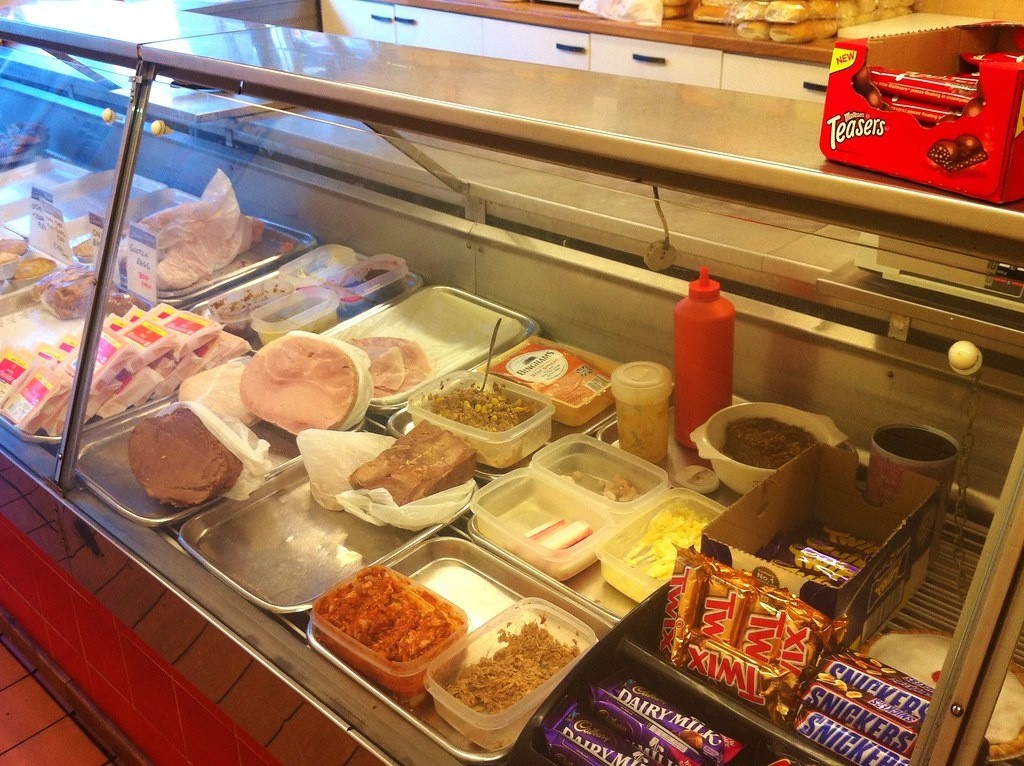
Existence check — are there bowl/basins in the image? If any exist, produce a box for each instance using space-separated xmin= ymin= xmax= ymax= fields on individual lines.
xmin=308 ymin=370 xmax=849 ymax=750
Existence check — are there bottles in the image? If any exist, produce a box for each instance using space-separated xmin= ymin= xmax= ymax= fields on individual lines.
xmin=673 ymin=264 xmax=737 ymax=449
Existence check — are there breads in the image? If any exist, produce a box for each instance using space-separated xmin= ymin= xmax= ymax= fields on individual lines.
xmin=659 ymin=541 xmax=833 ymax=729
xmin=660 ymin=0 xmax=916 ymax=44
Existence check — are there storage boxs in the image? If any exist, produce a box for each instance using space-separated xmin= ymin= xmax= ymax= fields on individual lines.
xmin=700 ymin=441 xmax=948 ymax=651
xmin=820 ymin=21 xmax=1024 ymax=205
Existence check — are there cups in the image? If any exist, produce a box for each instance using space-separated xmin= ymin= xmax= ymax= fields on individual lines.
xmin=864 ymin=422 xmax=960 ymax=520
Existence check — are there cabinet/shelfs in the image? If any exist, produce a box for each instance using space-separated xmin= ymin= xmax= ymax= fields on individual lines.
xmin=320 ymin=0 xmax=831 ymax=101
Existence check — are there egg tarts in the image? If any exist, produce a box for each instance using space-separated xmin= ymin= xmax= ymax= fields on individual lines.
xmin=71 ymin=238 xmax=94 ymax=263
xmin=0 ymin=238 xmax=56 ymax=290
